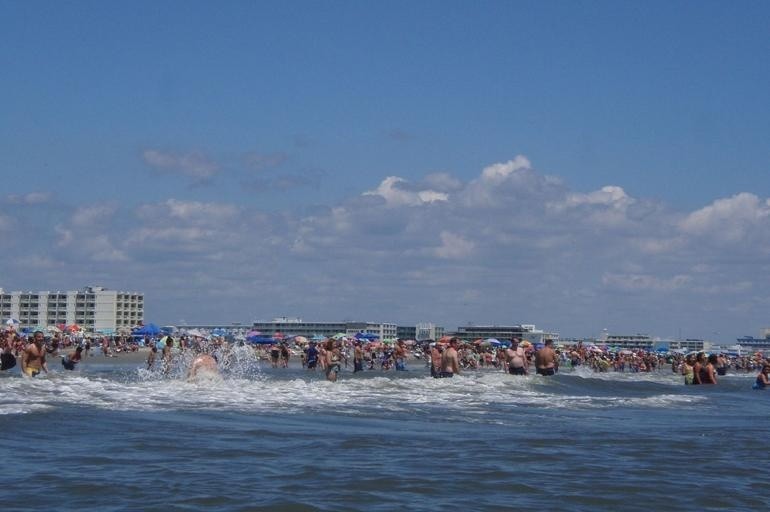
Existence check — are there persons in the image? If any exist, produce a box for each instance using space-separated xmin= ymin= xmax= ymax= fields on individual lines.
xmin=102 ymin=333 xmax=139 ymax=357
xmin=254 ymin=335 xmax=770 ymax=390
xmin=1 ymin=329 xmax=109 ymax=378
xmin=147 ymin=334 xmax=244 ymax=377
